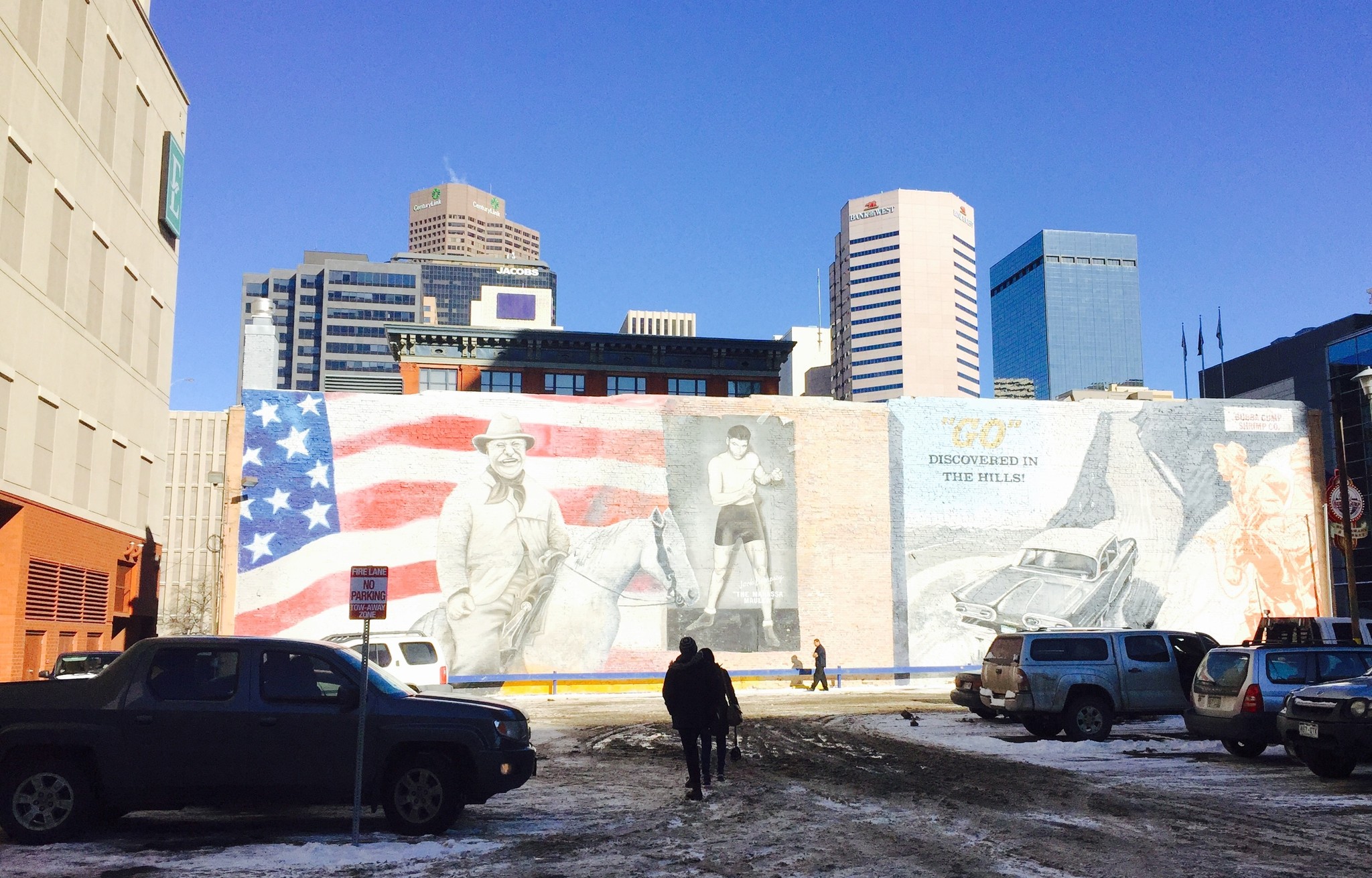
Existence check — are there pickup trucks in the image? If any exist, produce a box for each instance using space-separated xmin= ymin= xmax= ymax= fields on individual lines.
xmin=978 ymin=624 xmax=1221 ymax=741
xmin=1254 ymin=609 xmax=1372 ymax=644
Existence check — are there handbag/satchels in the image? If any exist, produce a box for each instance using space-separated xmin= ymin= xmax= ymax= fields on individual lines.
xmin=726 ymin=703 xmax=743 ymax=726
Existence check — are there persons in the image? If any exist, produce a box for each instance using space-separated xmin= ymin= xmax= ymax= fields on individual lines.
xmin=806 ymin=639 xmax=829 ymax=691
xmin=697 ymin=647 xmax=741 ymax=787
xmin=662 ymin=637 xmax=722 ymax=801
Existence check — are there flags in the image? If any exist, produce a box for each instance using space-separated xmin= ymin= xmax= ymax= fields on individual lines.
xmin=1197 ymin=320 xmax=1204 ymax=356
xmin=1181 ymin=330 xmax=1187 ymax=361
xmin=1216 ymin=310 xmax=1224 ymax=351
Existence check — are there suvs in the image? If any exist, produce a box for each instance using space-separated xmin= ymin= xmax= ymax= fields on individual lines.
xmin=319 ymin=630 xmax=452 ymax=692
xmin=1277 ymin=666 xmax=1372 ymax=777
xmin=1184 ymin=640 xmax=1372 ymax=757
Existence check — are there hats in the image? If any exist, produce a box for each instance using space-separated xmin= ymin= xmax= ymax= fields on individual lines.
xmin=471 ymin=421 xmax=536 ymax=454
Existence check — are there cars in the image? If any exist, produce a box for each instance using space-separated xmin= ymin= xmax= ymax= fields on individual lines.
xmin=38 ymin=651 xmax=126 ymax=682
xmin=950 ymin=668 xmax=1023 ymax=723
xmin=0 ymin=637 xmax=538 ymax=839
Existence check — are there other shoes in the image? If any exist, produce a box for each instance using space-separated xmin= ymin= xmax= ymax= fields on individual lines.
xmin=685 ymin=788 xmax=702 ymax=801
xmin=685 ymin=607 xmax=717 ymax=631
xmin=762 ymin=619 xmax=781 ymax=647
xmin=808 ymin=688 xmax=814 ymax=691
xmin=822 ymin=687 xmax=829 ymax=691
xmin=702 ymin=777 xmax=711 ymax=785
xmin=685 ymin=778 xmax=701 ymax=788
xmin=717 ymin=771 xmax=725 ymax=781
